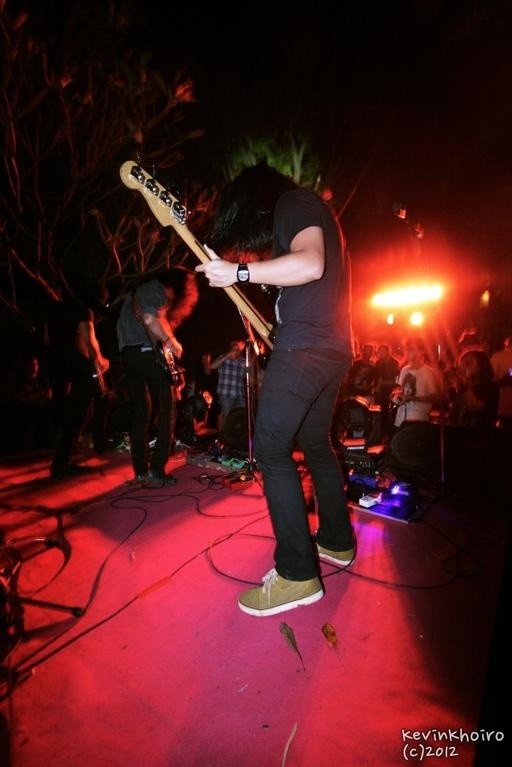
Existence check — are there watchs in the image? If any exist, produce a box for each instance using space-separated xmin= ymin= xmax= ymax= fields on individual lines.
xmin=237 ymin=263 xmax=249 ymax=283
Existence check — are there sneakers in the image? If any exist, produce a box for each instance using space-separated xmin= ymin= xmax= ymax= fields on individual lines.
xmin=310 ymin=539 xmax=359 ymax=567
xmin=50 ymin=440 xmax=179 ymax=489
xmin=237 ymin=567 xmax=328 ymax=617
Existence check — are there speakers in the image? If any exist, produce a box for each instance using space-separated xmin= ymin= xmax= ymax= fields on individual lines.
xmin=384 ymin=420 xmax=440 ymax=478
xmin=221 ymin=406 xmax=249 ymax=452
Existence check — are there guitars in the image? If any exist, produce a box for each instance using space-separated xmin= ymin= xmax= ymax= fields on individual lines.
xmin=118 ymin=162 xmax=275 ymax=371
xmin=161 ymin=338 xmax=180 ymax=385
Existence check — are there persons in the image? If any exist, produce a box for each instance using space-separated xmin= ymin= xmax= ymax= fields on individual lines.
xmin=48 ymin=281 xmax=113 ymax=480
xmin=192 ymin=165 xmax=357 ymax=619
xmin=112 ymin=264 xmax=200 ymax=487
